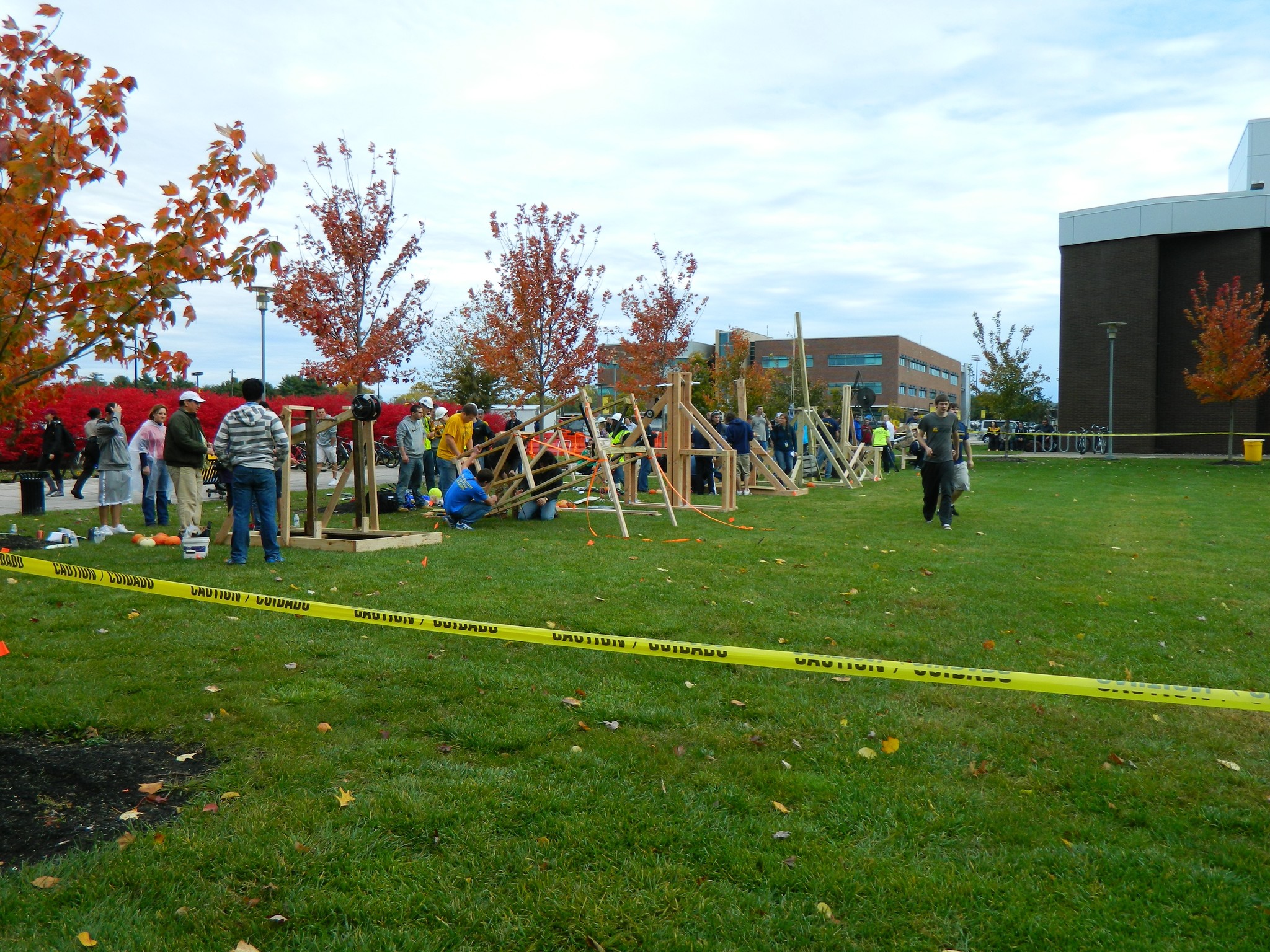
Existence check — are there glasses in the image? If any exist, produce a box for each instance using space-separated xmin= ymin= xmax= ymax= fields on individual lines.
xmin=100 ymin=412 xmax=103 ymax=414
xmin=779 ymin=418 xmax=785 ymax=419
xmin=712 ymin=411 xmax=720 ymax=416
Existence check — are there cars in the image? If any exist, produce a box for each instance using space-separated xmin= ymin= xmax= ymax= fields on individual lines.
xmin=1018 ymin=420 xmax=1042 ymax=434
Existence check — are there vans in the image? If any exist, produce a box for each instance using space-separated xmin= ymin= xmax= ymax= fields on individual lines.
xmin=977 ymin=420 xmax=1022 ymax=444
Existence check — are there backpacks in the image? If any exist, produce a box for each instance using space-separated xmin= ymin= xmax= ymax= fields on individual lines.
xmin=53 ymin=419 xmax=76 ymax=453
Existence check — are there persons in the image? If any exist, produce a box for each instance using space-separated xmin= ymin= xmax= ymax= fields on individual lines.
xmin=70 ymin=406 xmax=108 ymax=499
xmin=999 ymin=419 xmax=1014 ymax=452
xmin=312 ymin=407 xmax=340 ymax=487
xmin=93 ymin=402 xmax=136 ymax=535
xmin=987 ymin=421 xmax=1001 ymax=452
xmin=916 ymin=393 xmax=961 ymax=531
xmin=137 ymin=403 xmax=171 ymax=528
xmin=212 ymin=376 xmax=292 ymax=566
xmin=937 ymin=403 xmax=974 ymax=517
xmin=1033 ymin=417 xmax=1056 ymax=452
xmin=39 ymin=407 xmax=67 ymax=497
xmin=163 ymin=390 xmax=216 ymax=540
xmin=383 ymin=389 xmax=936 ymax=530
xmin=1015 ymin=421 xmax=1027 ymax=452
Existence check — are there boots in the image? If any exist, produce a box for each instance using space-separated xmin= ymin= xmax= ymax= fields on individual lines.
xmin=45 ymin=475 xmax=64 ymax=497
xmin=71 ymin=479 xmax=84 ymax=499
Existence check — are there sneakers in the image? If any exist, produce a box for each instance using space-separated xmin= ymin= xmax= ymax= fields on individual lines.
xmin=925 ymin=519 xmax=933 ymax=524
xmin=247 ymin=522 xmax=261 ymax=531
xmin=226 ymin=559 xmax=246 ymax=567
xmin=421 ymin=505 xmax=433 ymax=510
xmin=100 ymin=523 xmax=135 ymax=536
xmin=736 ymin=488 xmax=753 ymax=496
xmin=942 ymin=524 xmax=953 ymax=531
xmin=709 ymin=492 xmax=719 ymax=496
xmin=266 ymin=556 xmax=285 ymax=563
xmin=328 ymin=478 xmax=339 ymax=486
xmin=445 ymin=515 xmax=476 ymax=530
xmin=397 ymin=507 xmax=409 ymax=512
xmin=937 ymin=505 xmax=959 ymax=517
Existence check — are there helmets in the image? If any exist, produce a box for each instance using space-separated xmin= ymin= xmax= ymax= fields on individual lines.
xmin=598 ymin=413 xmax=625 ymax=423
xmin=419 ymin=396 xmax=448 ymax=420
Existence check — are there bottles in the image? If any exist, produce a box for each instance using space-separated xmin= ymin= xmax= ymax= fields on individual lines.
xmin=294 ymin=514 xmax=300 ymax=528
xmin=88 ymin=526 xmax=105 ymax=543
xmin=70 ymin=531 xmax=79 ymax=547
xmin=11 ymin=524 xmax=18 ymax=535
xmin=584 ymin=479 xmax=590 ymax=489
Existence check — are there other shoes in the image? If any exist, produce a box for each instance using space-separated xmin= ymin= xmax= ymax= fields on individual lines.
xmin=638 ymin=489 xmax=650 ymax=492
xmin=148 ymin=522 xmax=172 ymax=528
xmin=422 ymin=471 xmax=440 ymax=477
xmin=906 ymin=461 xmax=921 ymax=470
xmin=987 ymin=449 xmax=1027 ymax=452
xmin=177 ymin=532 xmax=197 ymax=540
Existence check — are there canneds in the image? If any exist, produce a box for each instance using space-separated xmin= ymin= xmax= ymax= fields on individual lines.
xmin=61 ymin=534 xmax=69 ymax=543
xmin=36 ymin=530 xmax=43 ymax=539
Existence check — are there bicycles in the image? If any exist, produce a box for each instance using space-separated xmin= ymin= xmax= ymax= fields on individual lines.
xmin=280 ymin=434 xmax=402 ymax=471
xmin=71 ymin=435 xmax=91 ymax=480
xmin=1076 ymin=426 xmax=1091 ymax=455
xmin=1091 ymin=423 xmax=1110 ymax=455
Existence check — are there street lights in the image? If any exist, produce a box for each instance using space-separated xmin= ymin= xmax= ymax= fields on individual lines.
xmin=1099 ymin=317 xmax=1125 ymax=458
xmin=611 ymin=356 xmax=620 ymax=418
xmin=245 ymin=282 xmax=282 ymax=403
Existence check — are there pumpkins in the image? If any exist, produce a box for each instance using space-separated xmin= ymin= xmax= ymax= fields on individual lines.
xmin=132 ymin=532 xmax=169 ymax=548
xmin=807 ymin=481 xmax=815 ymax=487
xmin=656 ymin=489 xmax=663 ymax=494
xmin=165 ymin=535 xmax=182 ymax=546
xmin=555 ymin=499 xmax=573 ymax=508
xmin=649 ymin=489 xmax=656 ymax=494
xmin=605 ymin=486 xmax=609 ymax=492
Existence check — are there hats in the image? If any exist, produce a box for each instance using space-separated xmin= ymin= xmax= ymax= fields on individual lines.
xmin=40 ymin=407 xmax=58 ymax=415
xmin=776 ymin=412 xmax=782 ymax=418
xmin=477 ymin=409 xmax=485 ymax=415
xmin=180 ymin=391 xmax=206 ymax=402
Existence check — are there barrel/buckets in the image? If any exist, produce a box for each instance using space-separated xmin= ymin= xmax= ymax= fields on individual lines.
xmin=1243 ymin=439 xmax=1263 ymax=461
xmin=180 ymin=537 xmax=210 ymax=559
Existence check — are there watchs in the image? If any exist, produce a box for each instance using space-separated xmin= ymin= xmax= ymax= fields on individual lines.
xmin=1047 ymin=436 xmax=1049 ymax=439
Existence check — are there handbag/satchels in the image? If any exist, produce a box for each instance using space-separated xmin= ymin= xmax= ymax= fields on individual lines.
xmin=365 ymin=488 xmax=398 ymax=515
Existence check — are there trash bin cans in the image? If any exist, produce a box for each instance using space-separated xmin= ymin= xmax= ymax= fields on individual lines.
xmin=16 ymin=472 xmax=50 ymax=514
